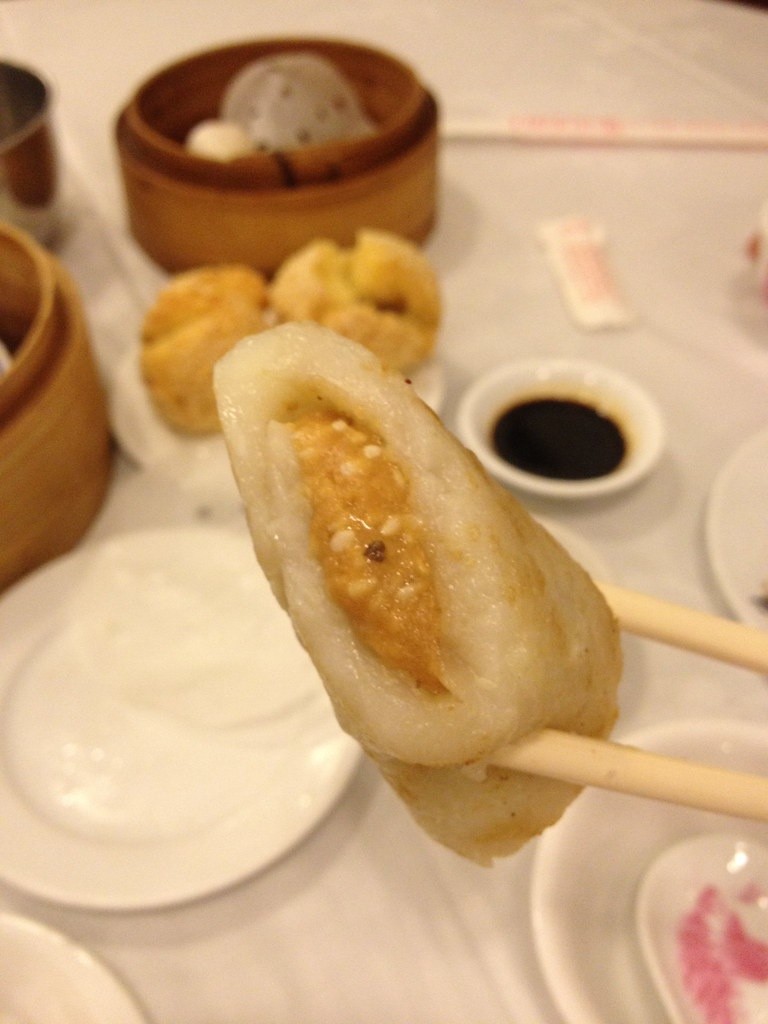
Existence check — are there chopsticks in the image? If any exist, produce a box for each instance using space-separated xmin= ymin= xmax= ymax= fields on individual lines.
xmin=491 ymin=579 xmax=766 ymax=822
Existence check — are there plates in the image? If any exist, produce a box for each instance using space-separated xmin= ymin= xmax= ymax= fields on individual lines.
xmin=1 ymin=526 xmax=365 ymax=910
xmin=2 ymin=911 xmax=146 ymax=1024
xmin=528 ymin=720 xmax=768 ymax=1024
xmin=708 ymin=427 xmax=767 ymax=634
xmin=109 ymin=321 xmax=443 ymax=498
xmin=459 ymin=357 xmax=668 ymax=500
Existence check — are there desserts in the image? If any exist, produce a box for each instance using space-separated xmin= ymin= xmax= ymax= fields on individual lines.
xmin=138 ymin=225 xmax=444 ymax=443
